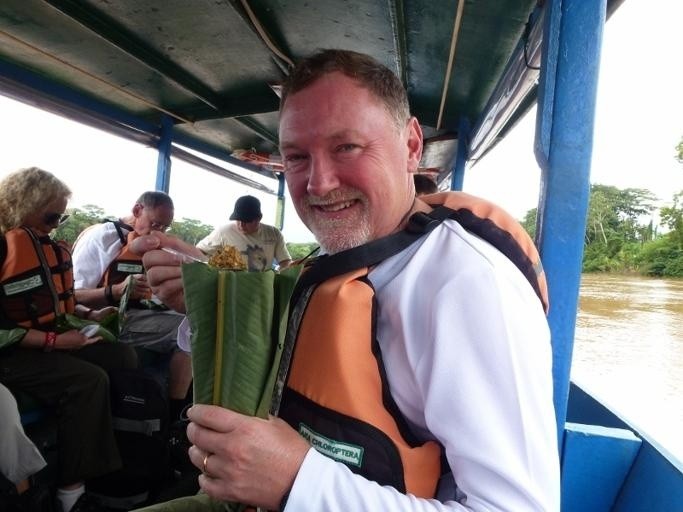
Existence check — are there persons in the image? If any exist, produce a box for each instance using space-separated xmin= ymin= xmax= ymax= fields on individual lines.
xmin=73 ymin=190 xmax=193 ymax=457
xmin=186 ymin=51 xmax=560 ymax=512
xmin=195 ymin=195 xmax=292 ymax=274
xmin=0 ymin=167 xmax=132 ymax=512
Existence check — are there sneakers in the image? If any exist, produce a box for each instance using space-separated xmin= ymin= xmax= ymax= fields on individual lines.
xmin=15 ymin=487 xmax=149 ymax=512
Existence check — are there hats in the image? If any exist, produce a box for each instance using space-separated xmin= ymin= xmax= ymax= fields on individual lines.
xmin=230 ymin=195 xmax=260 ymax=220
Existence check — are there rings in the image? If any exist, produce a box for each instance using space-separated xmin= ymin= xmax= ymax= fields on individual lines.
xmin=204 ymin=453 xmax=215 ymax=474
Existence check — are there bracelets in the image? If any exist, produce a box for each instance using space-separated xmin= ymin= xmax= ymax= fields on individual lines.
xmin=105 ymin=285 xmax=115 ymax=302
xmin=84 ymin=309 xmax=94 ymax=319
xmin=44 ymin=331 xmax=56 ymax=348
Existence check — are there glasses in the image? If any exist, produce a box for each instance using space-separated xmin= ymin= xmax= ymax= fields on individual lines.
xmin=152 ymin=223 xmax=172 ymax=234
xmin=43 ymin=213 xmax=70 ymax=225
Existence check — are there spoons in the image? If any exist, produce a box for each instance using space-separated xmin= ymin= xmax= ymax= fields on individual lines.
xmin=157 ymin=243 xmax=250 ymax=272
